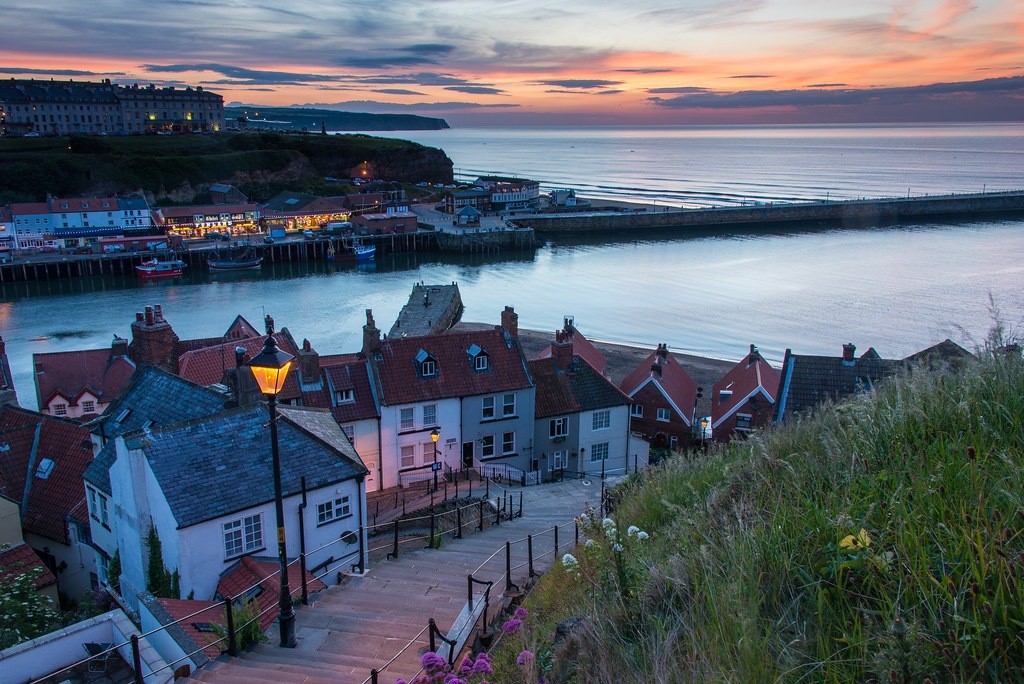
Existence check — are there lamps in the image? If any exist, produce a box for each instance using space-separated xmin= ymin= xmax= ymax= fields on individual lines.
xmin=82 ymin=440 xmax=97 ymax=451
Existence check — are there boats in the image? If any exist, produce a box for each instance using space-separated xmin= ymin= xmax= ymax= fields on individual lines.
xmin=353 ymin=245 xmax=376 ymax=265
xmin=134 ymin=253 xmax=188 ymax=276
xmin=205 ymin=233 xmax=264 ymax=269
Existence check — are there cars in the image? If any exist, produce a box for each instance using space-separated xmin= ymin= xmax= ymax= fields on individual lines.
xmin=324 ymin=176 xmax=337 ymax=182
xmin=416 ymin=182 xmax=428 ymax=187
xmin=433 ymin=183 xmax=491 ymax=192
xmin=350 ymin=177 xmax=366 ymax=186
xmin=204 ymin=232 xmax=221 ymax=240
xmin=303 ymin=228 xmax=313 ymax=235
xmin=263 ymin=236 xmax=274 ymax=244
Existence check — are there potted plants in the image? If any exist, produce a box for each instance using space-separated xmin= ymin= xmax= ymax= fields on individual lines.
xmin=554 ymin=435 xmax=566 ymax=443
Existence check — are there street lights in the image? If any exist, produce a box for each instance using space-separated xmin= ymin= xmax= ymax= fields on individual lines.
xmin=429 ymin=428 xmax=440 ymax=491
xmin=245 ymin=325 xmax=296 ymax=648
xmin=701 ymin=416 xmax=710 ymax=445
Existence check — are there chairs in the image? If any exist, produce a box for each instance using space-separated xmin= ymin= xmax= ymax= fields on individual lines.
xmin=82 ymin=642 xmax=120 ymax=673
